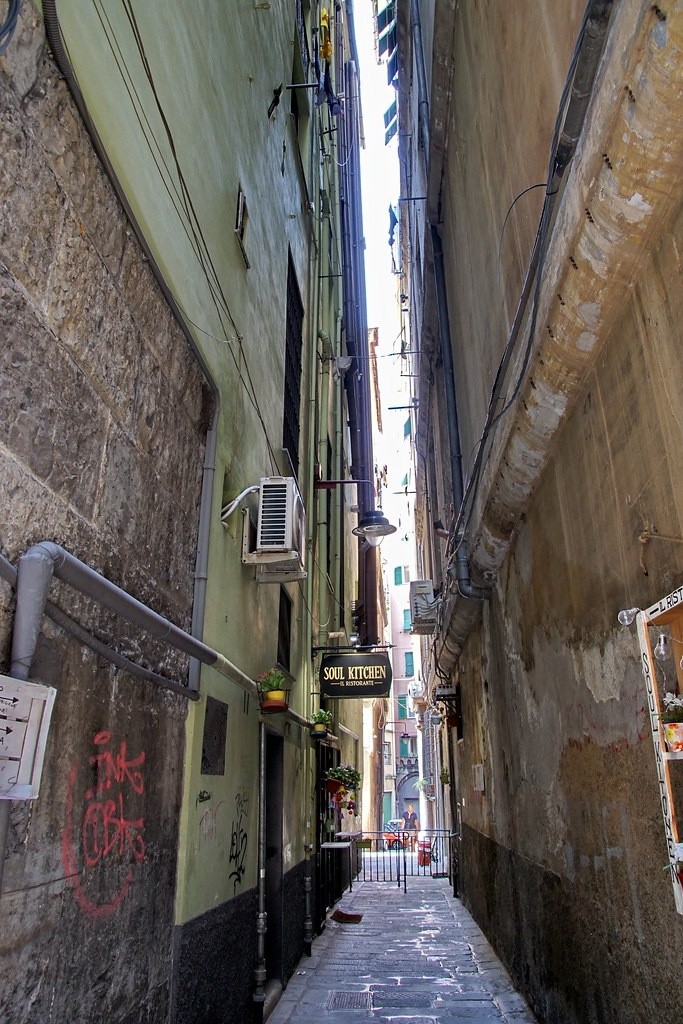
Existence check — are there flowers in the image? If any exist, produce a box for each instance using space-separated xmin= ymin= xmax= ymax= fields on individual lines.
xmin=326 ymin=762 xmax=362 ymax=819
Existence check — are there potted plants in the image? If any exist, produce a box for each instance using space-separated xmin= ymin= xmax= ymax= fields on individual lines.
xmin=256 ymin=667 xmax=293 ymax=701
xmin=440 ymin=767 xmax=449 ymax=783
xmin=309 ymin=709 xmax=334 ymax=731
xmin=430 ymin=711 xmax=441 ymax=725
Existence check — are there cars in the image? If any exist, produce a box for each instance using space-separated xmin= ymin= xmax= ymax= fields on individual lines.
xmin=388 ymin=819 xmax=406 ymax=829
xmin=384 ymin=824 xmax=408 ymax=848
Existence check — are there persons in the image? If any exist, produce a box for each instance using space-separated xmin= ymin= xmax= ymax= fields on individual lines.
xmin=400 ymin=804 xmax=419 ymax=853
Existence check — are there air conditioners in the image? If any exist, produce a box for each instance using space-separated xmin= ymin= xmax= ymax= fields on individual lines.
xmin=256 ymin=476 xmax=307 ymax=571
xmin=410 ymin=579 xmax=437 ymax=632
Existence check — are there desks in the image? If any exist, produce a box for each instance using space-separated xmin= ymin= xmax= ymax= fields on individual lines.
xmin=322 ymin=842 xmax=353 ymax=894
xmin=335 ymin=831 xmax=361 ymax=836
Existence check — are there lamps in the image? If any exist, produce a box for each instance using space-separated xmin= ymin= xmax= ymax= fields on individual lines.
xmin=317 ymin=480 xmax=397 ymax=547
xmin=352 ymin=639 xmax=361 ymax=647
xmin=385 ymin=721 xmax=413 ymax=744
xmin=349 ymin=632 xmax=358 ymax=641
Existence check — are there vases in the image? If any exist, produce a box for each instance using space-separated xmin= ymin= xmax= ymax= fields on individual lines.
xmin=429 ymin=784 xmax=434 ymax=792
xmin=328 ymin=778 xmax=342 ymax=794
